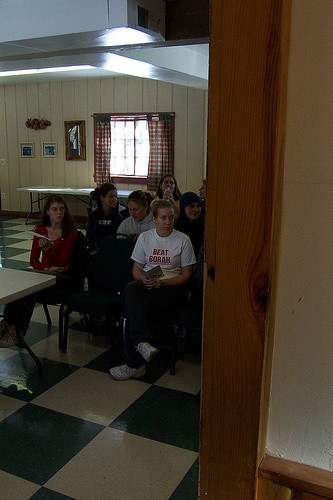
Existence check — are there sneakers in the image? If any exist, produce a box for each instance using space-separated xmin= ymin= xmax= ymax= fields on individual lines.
xmin=110 ymin=364 xmax=145 ymax=380
xmin=0 ymin=325 xmax=22 ymax=347
xmin=0 ymin=320 xmax=10 ymax=337
xmin=134 ymin=342 xmax=159 ymax=362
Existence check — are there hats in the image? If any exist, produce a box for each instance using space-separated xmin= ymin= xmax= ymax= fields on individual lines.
xmin=180 ymin=192 xmax=200 ymax=210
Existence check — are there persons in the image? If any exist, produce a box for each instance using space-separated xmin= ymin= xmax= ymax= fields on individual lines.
xmin=0 ymin=196 xmax=79 ymax=347
xmin=117 ymin=189 xmax=157 ymax=243
xmin=150 ymin=175 xmax=182 ymax=218
xmin=173 ymin=192 xmax=205 ymax=319
xmin=109 ymin=200 xmax=197 ymax=381
xmin=199 ymin=179 xmax=206 ymax=198
xmin=86 ymin=182 xmax=130 ymax=255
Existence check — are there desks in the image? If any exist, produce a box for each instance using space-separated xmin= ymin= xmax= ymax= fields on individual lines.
xmin=16 ymin=187 xmax=140 ymax=225
xmin=0 ymin=266 xmax=56 ymax=378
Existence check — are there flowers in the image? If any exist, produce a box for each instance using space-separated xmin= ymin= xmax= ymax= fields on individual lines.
xmin=25 ymin=117 xmax=51 ymax=130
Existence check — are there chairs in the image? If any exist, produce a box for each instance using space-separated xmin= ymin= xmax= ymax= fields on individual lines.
xmin=34 ymin=229 xmax=197 ymax=354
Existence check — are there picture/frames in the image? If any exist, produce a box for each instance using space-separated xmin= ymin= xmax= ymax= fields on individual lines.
xmin=41 ymin=141 xmax=57 ymax=158
xmin=65 ymin=120 xmax=85 ymax=160
xmin=19 ymin=142 xmax=34 ymax=157
